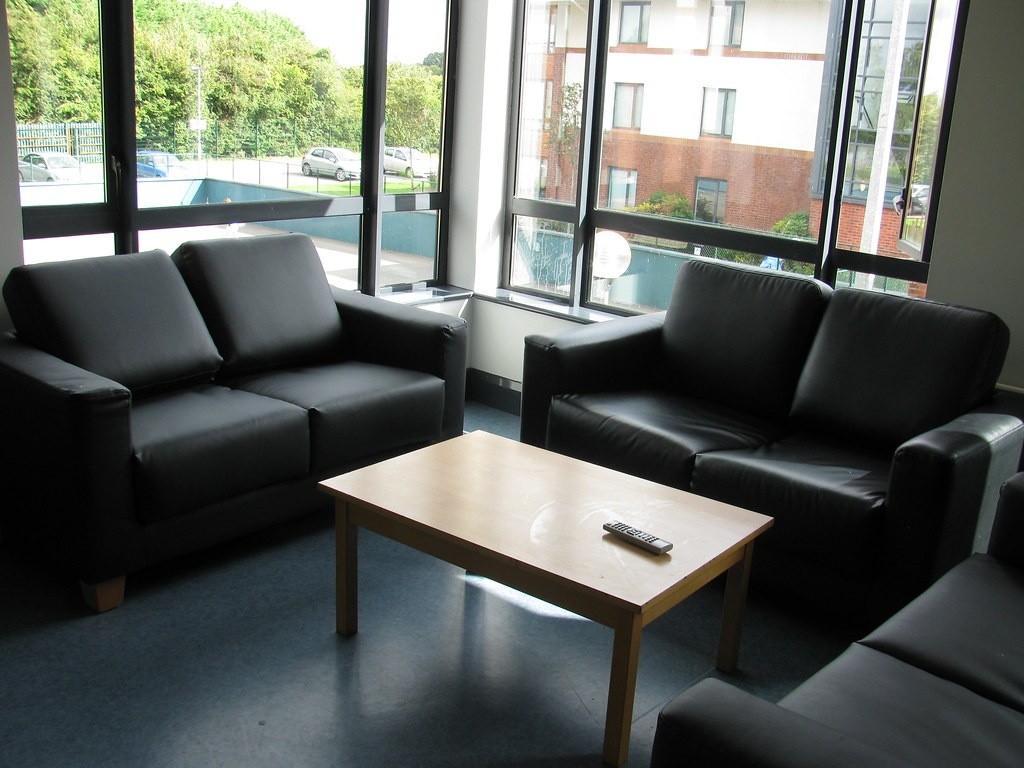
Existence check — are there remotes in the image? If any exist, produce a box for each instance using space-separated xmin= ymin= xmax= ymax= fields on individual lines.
xmin=603 ymin=520 xmax=673 ymax=556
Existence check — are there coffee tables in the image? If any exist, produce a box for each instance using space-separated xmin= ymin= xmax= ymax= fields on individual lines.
xmin=316 ymin=429 xmax=776 ymax=768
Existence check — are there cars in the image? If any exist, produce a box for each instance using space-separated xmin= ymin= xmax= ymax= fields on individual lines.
xmin=136 ymin=151 xmax=186 ymax=179
xmin=19 ymin=153 xmax=88 ymax=181
xmin=902 ymin=183 xmax=930 ymax=215
xmin=381 ymin=146 xmax=439 ymax=179
xmin=300 ymin=147 xmax=365 ymax=181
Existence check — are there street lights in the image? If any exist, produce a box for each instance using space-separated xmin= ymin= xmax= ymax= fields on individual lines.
xmin=189 ymin=65 xmax=206 ymax=159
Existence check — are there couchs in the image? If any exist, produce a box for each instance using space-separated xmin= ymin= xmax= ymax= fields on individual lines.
xmin=519 ymin=255 xmax=1024 ymax=632
xmin=648 ymin=471 xmax=1024 ymax=768
xmin=0 ymin=232 xmax=468 ymax=614
